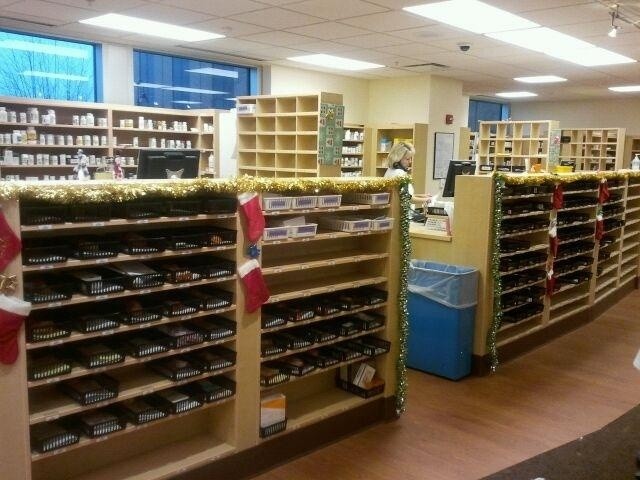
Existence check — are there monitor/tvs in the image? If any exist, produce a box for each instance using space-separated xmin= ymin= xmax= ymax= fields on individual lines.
xmin=136 ymin=147 xmax=200 ymax=180
xmin=442 ymin=160 xmax=476 ymax=198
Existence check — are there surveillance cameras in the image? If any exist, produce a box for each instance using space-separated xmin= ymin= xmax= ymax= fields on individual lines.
xmin=458 ymin=43 xmax=471 ymax=52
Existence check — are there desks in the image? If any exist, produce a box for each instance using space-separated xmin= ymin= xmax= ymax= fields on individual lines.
xmin=408 ymin=217 xmax=453 ymax=264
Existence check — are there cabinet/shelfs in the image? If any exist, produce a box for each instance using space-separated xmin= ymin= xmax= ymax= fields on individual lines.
xmin=580 ymin=132 xmax=640 ymax=171
xmin=0 ymin=96 xmax=229 ymax=182
xmin=340 ymin=123 xmax=374 ymax=177
xmin=520 ymin=135 xmax=544 ymax=169
xmin=235 ymin=92 xmax=345 ymax=178
xmin=372 ymin=122 xmax=428 ymax=209
xmin=543 ymin=128 xmax=634 ymax=172
xmin=458 ymin=127 xmax=512 ymax=171
xmin=0 ymin=176 xmax=403 ymax=480
xmin=476 ymin=120 xmax=561 ymax=177
xmin=445 ymin=170 xmax=640 ymax=356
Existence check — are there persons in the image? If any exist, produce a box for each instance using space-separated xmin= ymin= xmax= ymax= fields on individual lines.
xmin=385 ymin=141 xmax=432 ymax=220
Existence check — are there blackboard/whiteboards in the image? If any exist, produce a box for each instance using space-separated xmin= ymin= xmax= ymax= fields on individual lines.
xmin=434 ymin=131 xmax=455 ymax=180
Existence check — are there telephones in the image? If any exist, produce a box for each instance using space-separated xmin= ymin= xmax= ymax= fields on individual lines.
xmin=393 ymin=162 xmax=428 ymax=225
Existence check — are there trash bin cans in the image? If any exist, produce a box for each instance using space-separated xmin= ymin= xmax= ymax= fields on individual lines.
xmin=406 ymin=260 xmax=479 ymax=382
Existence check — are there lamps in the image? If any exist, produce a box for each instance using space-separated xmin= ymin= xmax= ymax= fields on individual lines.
xmin=608 ymin=16 xmax=620 ymax=40
